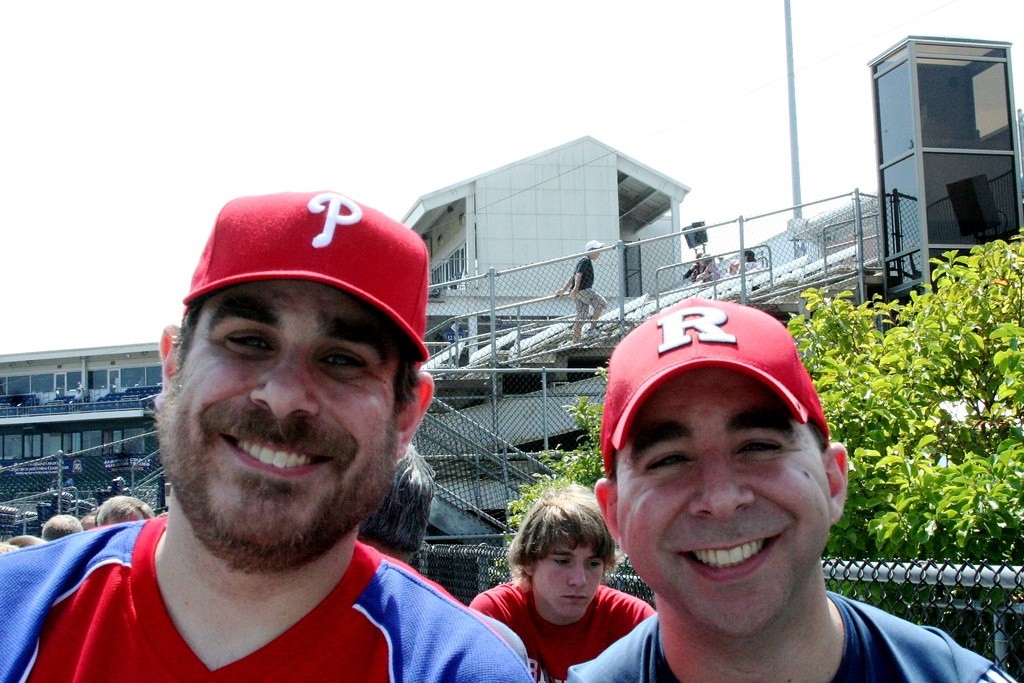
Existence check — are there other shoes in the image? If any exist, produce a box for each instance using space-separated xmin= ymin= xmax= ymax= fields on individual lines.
xmin=587 ymin=328 xmax=601 ymax=334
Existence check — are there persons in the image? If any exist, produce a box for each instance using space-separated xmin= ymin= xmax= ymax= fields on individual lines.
xmin=467 ymin=483 xmax=660 ymax=682
xmin=0 ymin=189 xmax=539 ymax=682
xmin=357 ymin=444 xmax=436 ymax=565
xmin=681 ymin=249 xmax=760 ymax=285
xmin=68 ymin=388 xmax=84 ymax=411
xmin=0 ymin=473 xmax=159 ymax=557
xmin=562 ymin=297 xmax=1016 ymax=682
xmin=555 ymin=240 xmax=607 ymax=346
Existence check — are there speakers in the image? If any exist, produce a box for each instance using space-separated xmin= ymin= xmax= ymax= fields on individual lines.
xmin=945 ymin=173 xmax=1002 ymax=236
xmin=682 ymin=222 xmax=707 ymax=249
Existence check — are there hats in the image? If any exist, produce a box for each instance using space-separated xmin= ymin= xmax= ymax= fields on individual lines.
xmin=182 ymin=190 xmax=431 ymax=371
xmin=586 ymin=240 xmax=605 ymax=252
xmin=599 ymin=299 xmax=829 ymax=480
